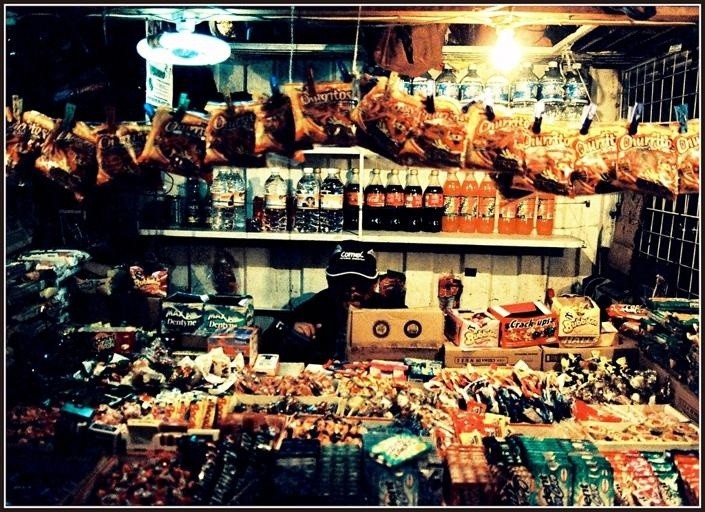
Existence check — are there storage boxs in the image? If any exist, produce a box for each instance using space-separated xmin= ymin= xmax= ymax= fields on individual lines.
xmin=444 ymin=342 xmax=542 ymax=371
xmin=345 ymin=304 xmax=445 ymax=348
xmin=540 ymin=338 xmax=640 ymax=373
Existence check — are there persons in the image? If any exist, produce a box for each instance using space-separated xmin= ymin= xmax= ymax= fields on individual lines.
xmin=265 ymin=245 xmax=407 ymax=371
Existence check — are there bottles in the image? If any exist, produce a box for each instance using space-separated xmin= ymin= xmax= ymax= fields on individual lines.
xmin=261 ymin=166 xmax=345 ymax=234
xmin=347 ymin=167 xmax=557 ymax=232
xmin=226 ymin=168 xmax=246 ymax=228
xmin=399 ymin=61 xmax=592 ymax=117
xmin=213 ymin=168 xmax=233 ymax=231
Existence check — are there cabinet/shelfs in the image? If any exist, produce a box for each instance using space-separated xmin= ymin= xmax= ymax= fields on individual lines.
xmin=135 ymin=48 xmax=585 ymax=248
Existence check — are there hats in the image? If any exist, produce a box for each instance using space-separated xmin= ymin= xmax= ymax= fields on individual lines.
xmin=325 ymin=240 xmax=379 ymax=280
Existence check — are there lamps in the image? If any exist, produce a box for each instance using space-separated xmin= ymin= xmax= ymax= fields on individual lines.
xmin=136 ymin=19 xmax=231 ymax=66
